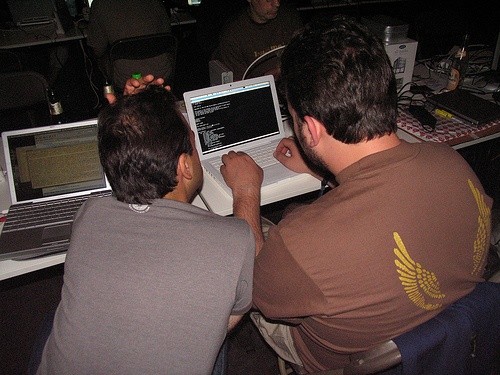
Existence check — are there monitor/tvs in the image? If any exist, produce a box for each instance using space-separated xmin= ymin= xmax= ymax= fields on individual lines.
xmin=87 ymin=0 xmax=93 ymax=9
xmin=187 ymin=0 xmax=202 ymax=7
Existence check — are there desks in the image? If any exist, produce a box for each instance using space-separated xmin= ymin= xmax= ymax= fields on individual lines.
xmin=0 ymin=60 xmax=500 ymax=281
xmin=170 ymin=12 xmax=196 ymax=26
xmin=295 ymin=0 xmax=396 ymax=11
xmin=0 ymin=15 xmax=85 ymax=50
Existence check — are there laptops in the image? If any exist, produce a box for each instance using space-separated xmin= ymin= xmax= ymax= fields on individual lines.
xmin=183 ymin=74 xmax=302 ymax=195
xmin=0 ymin=118 xmax=114 ymax=260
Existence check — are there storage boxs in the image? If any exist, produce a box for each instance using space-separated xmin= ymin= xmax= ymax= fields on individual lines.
xmin=383 ymin=37 xmax=418 ymax=98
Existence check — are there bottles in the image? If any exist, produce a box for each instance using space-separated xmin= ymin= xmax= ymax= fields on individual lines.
xmin=45 ymin=86 xmax=67 ymax=126
xmin=98 ymin=70 xmax=119 ymax=116
xmin=131 ymin=72 xmax=142 ymax=79
xmin=446 ymin=32 xmax=473 ymax=90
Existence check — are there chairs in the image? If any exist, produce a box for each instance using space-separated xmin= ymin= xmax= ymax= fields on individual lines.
xmin=414 ymin=11 xmax=454 ymax=59
xmin=278 ymin=272 xmax=500 ymax=375
xmin=100 ymin=33 xmax=178 ymax=98
xmin=0 ymin=70 xmax=53 ymax=127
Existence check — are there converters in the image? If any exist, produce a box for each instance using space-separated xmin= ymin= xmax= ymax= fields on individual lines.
xmin=408 ymin=105 xmax=437 ymax=126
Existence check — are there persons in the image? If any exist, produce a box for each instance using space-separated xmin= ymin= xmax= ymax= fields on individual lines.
xmin=221 ymin=14 xmax=493 ymax=375
xmin=86 ymin=0 xmax=173 ymax=89
xmin=28 ymin=74 xmax=255 ymax=375
xmin=212 ymin=0 xmax=307 ymax=83
